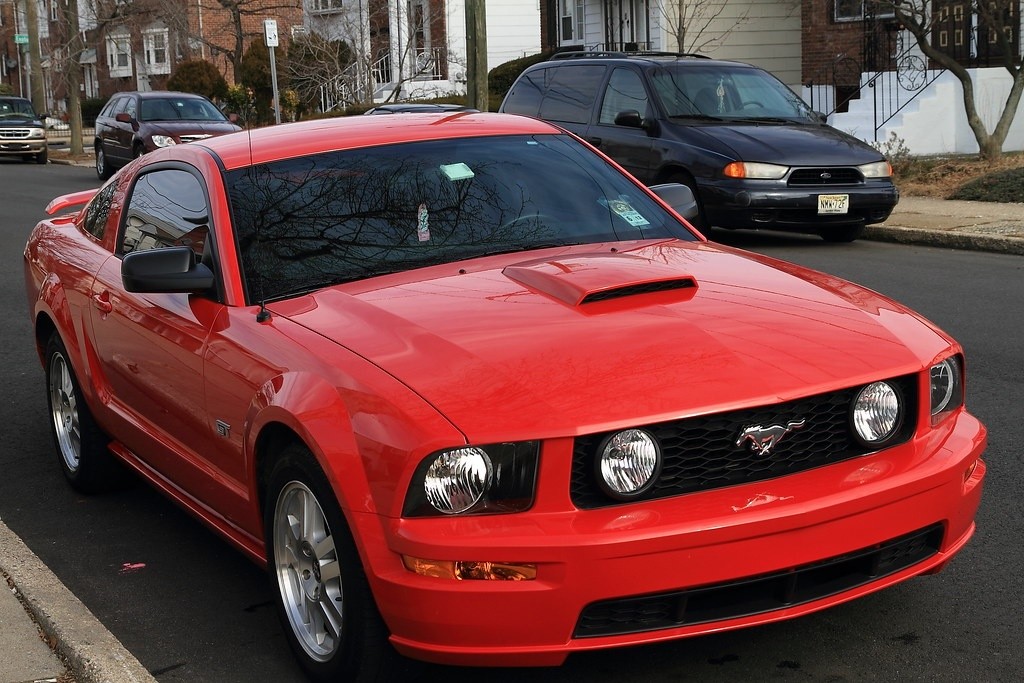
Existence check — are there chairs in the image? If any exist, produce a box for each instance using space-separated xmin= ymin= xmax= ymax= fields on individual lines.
xmin=692 ymin=88 xmax=721 ymax=115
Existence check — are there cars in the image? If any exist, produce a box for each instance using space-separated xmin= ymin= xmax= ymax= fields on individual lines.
xmin=365 ymin=102 xmax=482 ymax=115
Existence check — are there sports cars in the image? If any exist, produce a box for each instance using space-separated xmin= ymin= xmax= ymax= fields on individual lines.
xmin=22 ymin=110 xmax=990 ymax=683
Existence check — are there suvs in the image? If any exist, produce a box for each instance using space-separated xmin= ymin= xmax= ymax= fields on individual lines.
xmin=94 ymin=91 xmax=244 ymax=180
xmin=494 ymin=48 xmax=901 ymax=244
xmin=0 ymin=97 xmax=48 ymax=165
